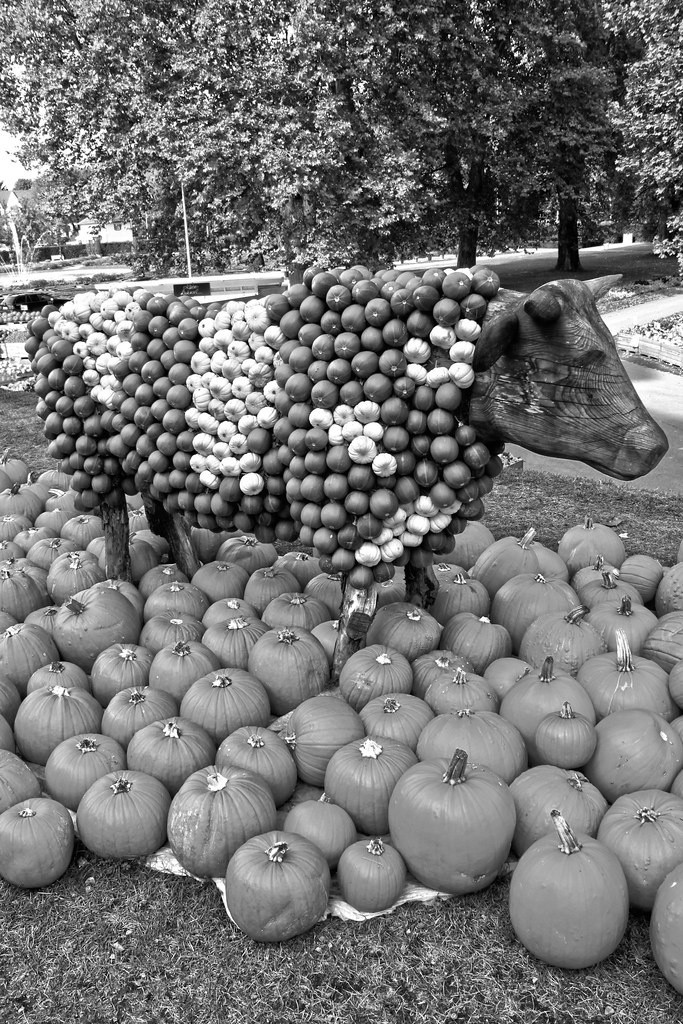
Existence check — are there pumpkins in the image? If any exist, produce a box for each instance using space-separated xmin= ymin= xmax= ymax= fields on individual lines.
xmin=0 ymin=264 xmax=683 ymax=995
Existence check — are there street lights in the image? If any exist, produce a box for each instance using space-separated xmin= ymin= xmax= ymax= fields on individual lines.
xmin=180 ymin=172 xmax=192 ymax=278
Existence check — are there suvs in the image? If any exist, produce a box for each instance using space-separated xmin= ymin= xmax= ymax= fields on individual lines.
xmin=2 ymin=292 xmax=72 ymax=314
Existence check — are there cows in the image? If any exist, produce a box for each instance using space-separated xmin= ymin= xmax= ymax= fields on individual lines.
xmin=26 ymin=275 xmax=668 ymax=610
